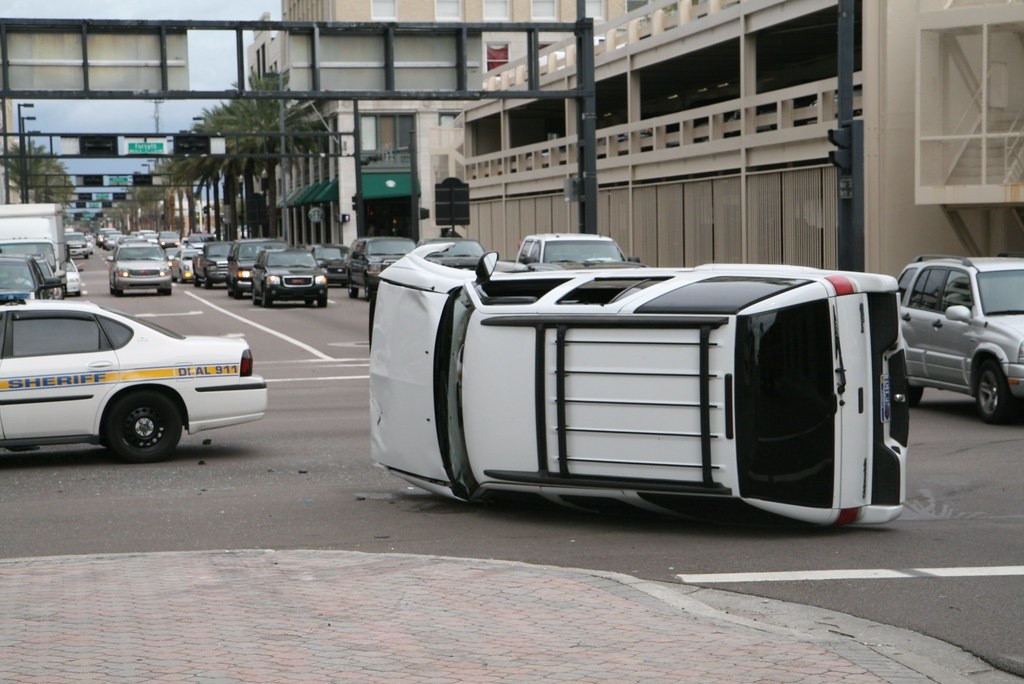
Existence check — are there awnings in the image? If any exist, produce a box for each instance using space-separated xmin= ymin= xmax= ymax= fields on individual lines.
xmin=276 ymin=177 xmax=340 ymax=208
xmin=360 ymin=172 xmax=421 ymax=199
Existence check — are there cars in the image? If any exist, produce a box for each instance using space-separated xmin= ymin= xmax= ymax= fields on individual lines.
xmin=417 ymin=239 xmax=487 ymax=269
xmin=0 ymin=292 xmax=270 ymax=461
xmin=170 ymin=249 xmax=200 ymax=283
xmin=107 ymin=244 xmax=175 ymax=297
xmin=304 ymin=245 xmax=351 ymax=285
xmin=33 ymin=254 xmax=65 ymax=300
xmin=89 ymin=228 xmax=219 ymax=258
xmin=66 ymin=258 xmax=85 ymax=295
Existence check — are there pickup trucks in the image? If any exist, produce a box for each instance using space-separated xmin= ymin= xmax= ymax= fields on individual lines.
xmin=192 ymin=242 xmax=235 ymax=289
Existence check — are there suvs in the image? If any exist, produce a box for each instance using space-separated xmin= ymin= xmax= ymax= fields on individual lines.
xmin=252 ymin=248 xmax=328 ymax=308
xmin=0 ymin=255 xmax=61 ymax=300
xmin=345 ymin=236 xmax=416 ymax=298
xmin=65 ymin=232 xmax=88 ymax=259
xmin=227 ymin=241 xmax=290 ymax=299
xmin=515 ymin=233 xmax=630 ymax=265
xmin=892 ymin=253 xmax=1024 ymax=423
xmin=367 ymin=239 xmax=911 ymax=528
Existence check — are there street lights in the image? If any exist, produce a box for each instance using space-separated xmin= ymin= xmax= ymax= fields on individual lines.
xmin=18 ymin=102 xmax=42 ymax=203
xmin=140 ymin=117 xmax=211 ymax=234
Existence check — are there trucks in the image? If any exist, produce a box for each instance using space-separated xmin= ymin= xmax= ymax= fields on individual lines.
xmin=0 ymin=203 xmax=68 ymax=294
